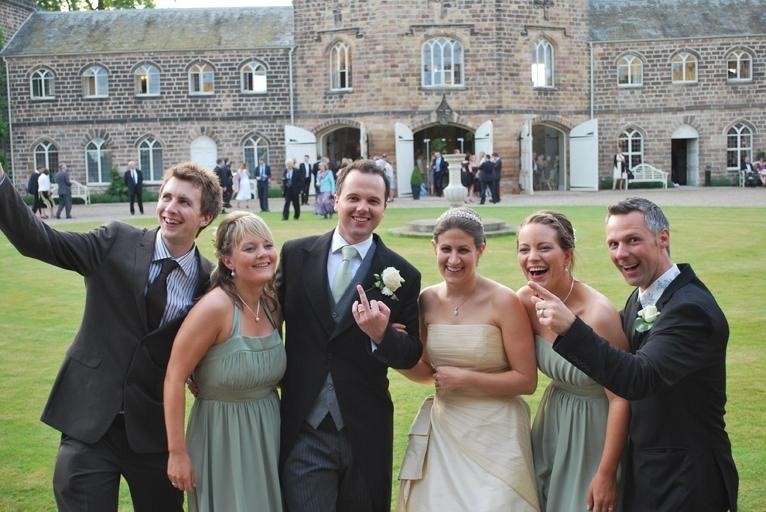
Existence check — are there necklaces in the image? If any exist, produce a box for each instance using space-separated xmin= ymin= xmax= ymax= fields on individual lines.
xmin=232 ymin=287 xmax=262 ymax=323
xmin=563 ymin=274 xmax=575 ymax=304
xmin=442 ymin=275 xmax=481 ymax=316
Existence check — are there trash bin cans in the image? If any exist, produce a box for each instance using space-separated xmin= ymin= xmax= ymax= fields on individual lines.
xmin=705 ymin=166 xmax=711 ymax=185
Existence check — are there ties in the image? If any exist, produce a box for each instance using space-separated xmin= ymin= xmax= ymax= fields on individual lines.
xmin=145 ymin=258 xmax=179 ymax=333
xmin=332 ymin=246 xmax=357 ymax=304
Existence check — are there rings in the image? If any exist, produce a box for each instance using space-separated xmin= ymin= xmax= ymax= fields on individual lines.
xmin=608 ymin=504 xmax=613 ymax=511
xmin=172 ymin=482 xmax=177 ymax=487
xmin=539 ymin=309 xmax=544 ymax=317
xmin=357 ymin=306 xmax=364 ymax=313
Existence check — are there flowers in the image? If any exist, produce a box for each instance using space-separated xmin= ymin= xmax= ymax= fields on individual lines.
xmin=632 ymin=302 xmax=663 ymax=339
xmin=362 ymin=264 xmax=406 ymax=302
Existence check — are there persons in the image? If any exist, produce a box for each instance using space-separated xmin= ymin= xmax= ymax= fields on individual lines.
xmin=511 ymin=209 xmax=629 ymax=512
xmin=526 ymin=196 xmax=739 ymax=512
xmin=0 ymin=161 xmax=219 ymax=512
xmin=389 ymin=207 xmax=542 ymax=512
xmin=612 ymin=148 xmax=627 ymax=191
xmin=186 ymin=158 xmax=423 ymax=512
xmin=161 ymin=209 xmax=287 ymax=512
xmin=741 ymin=155 xmax=762 ymax=187
xmin=756 ymin=157 xmax=766 ymax=187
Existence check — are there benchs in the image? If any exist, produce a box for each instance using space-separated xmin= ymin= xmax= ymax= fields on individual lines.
xmin=738 ymin=159 xmax=765 ymax=187
xmin=623 ymin=163 xmax=670 ymax=190
xmin=48 ymin=179 xmax=92 ymax=207
